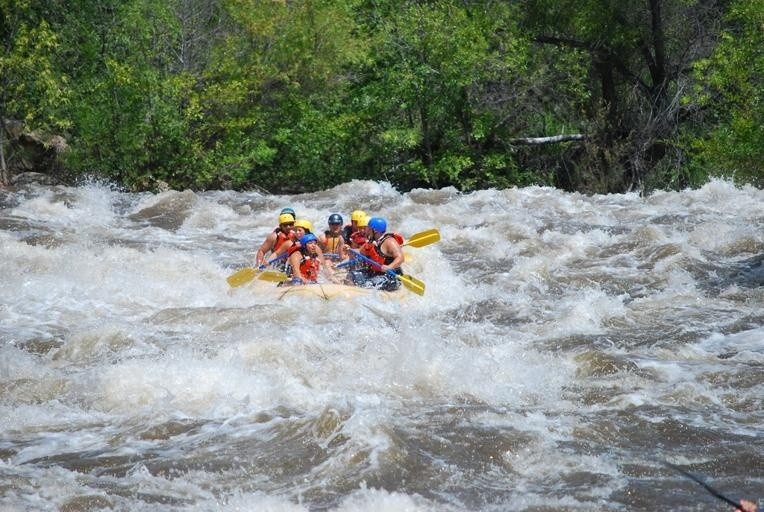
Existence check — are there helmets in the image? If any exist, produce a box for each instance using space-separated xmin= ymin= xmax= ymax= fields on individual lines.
xmin=300 ymin=234 xmax=317 ymax=246
xmin=293 ymin=219 xmax=312 ymax=233
xmin=351 ymin=210 xmax=371 ymax=227
xmin=278 ymin=208 xmax=296 ymax=226
xmin=368 ymin=218 xmax=386 ymax=233
xmin=328 ymin=214 xmax=342 ymax=224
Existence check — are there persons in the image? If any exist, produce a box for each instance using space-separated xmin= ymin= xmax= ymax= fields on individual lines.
xmin=256 ymin=209 xmax=403 ymax=291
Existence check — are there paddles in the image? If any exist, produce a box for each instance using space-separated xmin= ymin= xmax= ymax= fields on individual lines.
xmin=323 ymin=252 xmax=414 ymax=261
xmin=335 ymin=228 xmax=441 ymax=268
xmin=344 ymin=247 xmax=425 ymax=296
xmin=227 ymin=254 xmax=288 ymax=287
xmin=259 ymin=271 xmax=317 ymax=283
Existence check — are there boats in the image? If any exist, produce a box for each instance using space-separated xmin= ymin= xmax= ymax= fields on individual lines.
xmin=273 ymin=284 xmax=411 ymax=306
